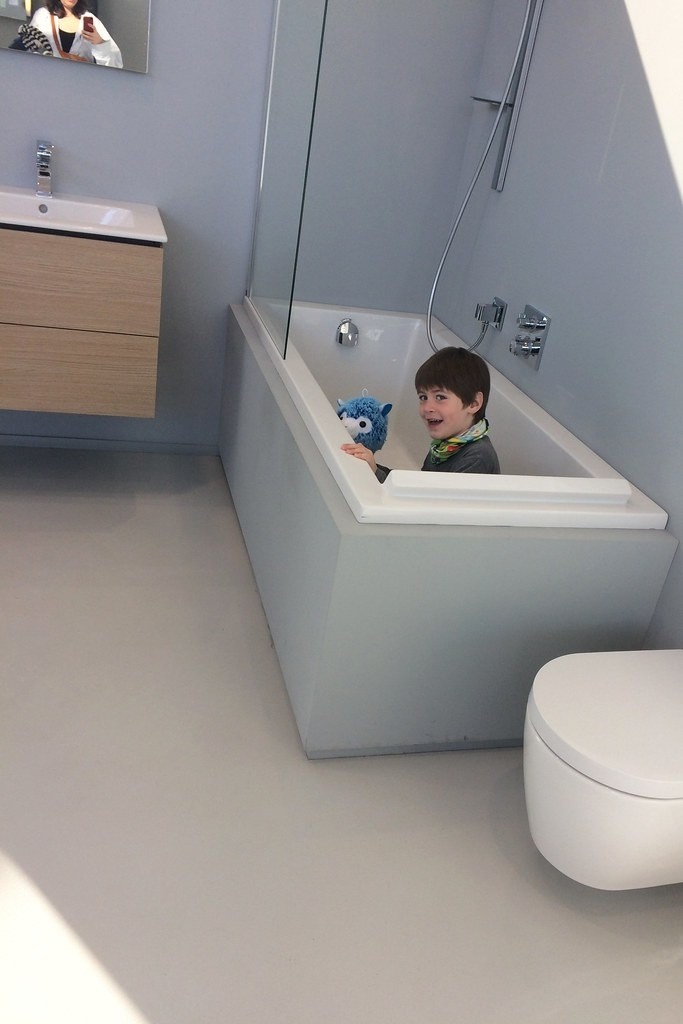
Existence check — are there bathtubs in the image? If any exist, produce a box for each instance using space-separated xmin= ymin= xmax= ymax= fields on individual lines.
xmin=219 ymin=295 xmax=683 ymax=762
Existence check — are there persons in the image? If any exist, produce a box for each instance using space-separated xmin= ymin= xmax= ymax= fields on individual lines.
xmin=30 ymin=0 xmax=123 ymax=68
xmin=341 ymin=346 xmax=500 ymax=484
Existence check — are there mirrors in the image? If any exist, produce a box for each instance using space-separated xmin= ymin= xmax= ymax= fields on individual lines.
xmin=0 ymin=1 xmax=153 ymax=73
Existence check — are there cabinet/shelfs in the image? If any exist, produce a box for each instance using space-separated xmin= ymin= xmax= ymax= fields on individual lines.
xmin=0 ymin=230 xmax=163 ymax=421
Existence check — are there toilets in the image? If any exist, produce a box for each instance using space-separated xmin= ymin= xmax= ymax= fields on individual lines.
xmin=523 ymin=647 xmax=683 ymax=890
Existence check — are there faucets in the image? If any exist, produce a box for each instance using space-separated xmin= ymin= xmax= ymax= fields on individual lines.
xmin=36 ymin=139 xmax=55 ymax=199
xmin=336 ymin=318 xmax=360 ymax=348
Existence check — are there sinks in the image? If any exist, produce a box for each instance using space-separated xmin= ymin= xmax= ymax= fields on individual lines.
xmin=0 ymin=184 xmax=168 ymax=244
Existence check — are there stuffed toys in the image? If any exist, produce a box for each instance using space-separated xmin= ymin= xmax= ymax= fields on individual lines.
xmin=337 ymin=396 xmax=392 ymax=454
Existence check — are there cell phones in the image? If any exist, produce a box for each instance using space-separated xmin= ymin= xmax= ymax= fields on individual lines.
xmin=84 ymin=17 xmax=93 ymax=33
